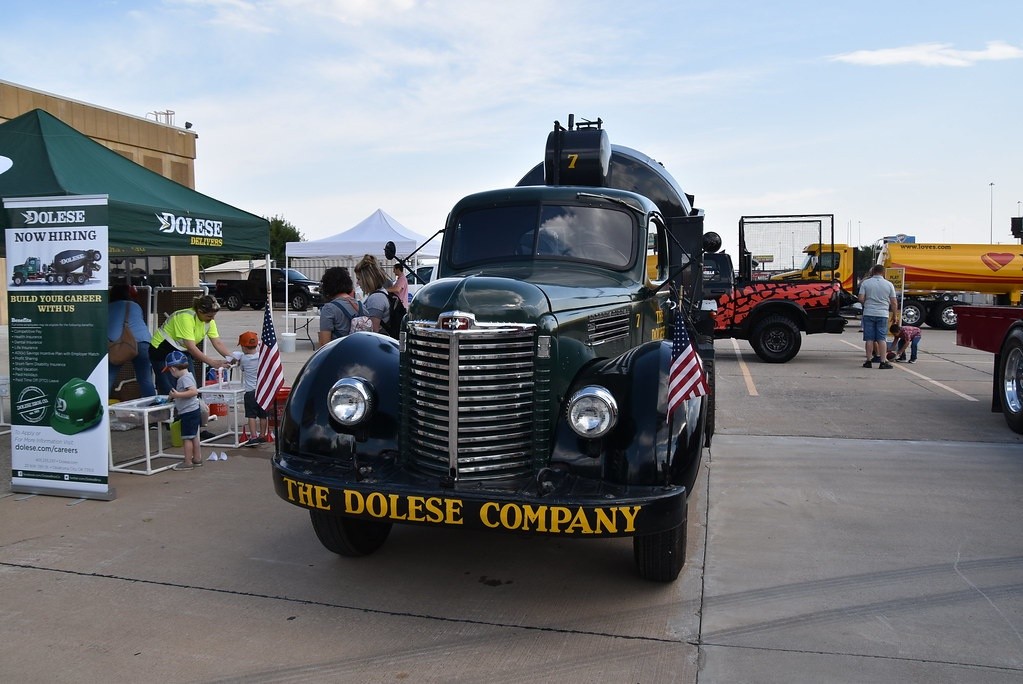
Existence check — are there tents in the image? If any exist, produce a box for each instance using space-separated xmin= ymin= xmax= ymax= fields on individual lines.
xmin=286 ymin=209 xmax=440 ymax=335
xmin=0 ymin=108 xmax=271 ymax=455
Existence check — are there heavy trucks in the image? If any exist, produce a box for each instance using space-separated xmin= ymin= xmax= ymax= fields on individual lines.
xmin=768 ymin=243 xmax=1023 ymax=330
xmin=12 ymin=250 xmax=102 ymax=286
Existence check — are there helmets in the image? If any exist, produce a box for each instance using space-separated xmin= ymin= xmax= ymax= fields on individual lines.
xmin=50 ymin=378 xmax=104 ymax=435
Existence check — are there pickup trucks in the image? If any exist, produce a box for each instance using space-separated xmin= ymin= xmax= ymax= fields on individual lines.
xmin=703 ymin=251 xmax=848 ymax=363
xmin=215 ymin=267 xmax=330 ymax=311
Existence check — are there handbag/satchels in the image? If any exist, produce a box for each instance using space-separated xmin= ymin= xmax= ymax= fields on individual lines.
xmin=198 ymin=397 xmax=209 ymax=427
xmin=108 ymin=322 xmax=138 ymax=366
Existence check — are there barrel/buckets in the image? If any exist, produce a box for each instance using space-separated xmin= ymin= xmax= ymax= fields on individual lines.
xmin=268 ymin=387 xmax=291 ymax=426
xmin=206 ymin=381 xmax=227 ymax=416
xmin=281 ymin=332 xmax=296 ymax=353
xmin=169 ymin=421 xmax=184 ymax=447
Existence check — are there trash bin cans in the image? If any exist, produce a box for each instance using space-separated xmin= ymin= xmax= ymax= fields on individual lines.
xmin=280 ymin=333 xmax=297 ymax=353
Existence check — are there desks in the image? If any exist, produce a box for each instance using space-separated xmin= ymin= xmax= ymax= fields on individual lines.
xmin=282 ymin=314 xmax=320 ymax=351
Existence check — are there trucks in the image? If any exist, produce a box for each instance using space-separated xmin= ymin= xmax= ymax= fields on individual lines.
xmin=272 ymin=112 xmax=720 ymax=584
xmin=954 ymin=306 xmax=1023 ymax=434
xmin=353 ymin=264 xmax=438 ymax=304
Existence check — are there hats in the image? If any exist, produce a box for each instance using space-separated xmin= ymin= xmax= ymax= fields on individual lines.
xmin=237 ymin=331 xmax=259 ymax=347
xmin=162 ymin=351 xmax=189 ymax=373
xmin=869 ymin=267 xmax=874 ymax=276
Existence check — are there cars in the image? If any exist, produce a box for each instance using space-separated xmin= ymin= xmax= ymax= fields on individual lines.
xmin=199 ymin=279 xmax=227 ymax=308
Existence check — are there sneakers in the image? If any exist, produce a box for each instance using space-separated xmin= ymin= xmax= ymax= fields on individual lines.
xmin=871 ymin=356 xmax=881 ymax=362
xmin=245 ymin=437 xmax=266 ymax=446
xmin=173 ymin=462 xmax=194 ymax=471
xmin=183 ymin=458 xmax=202 ymax=467
xmin=909 ymin=357 xmax=917 ymax=363
xmin=872 ymin=351 xmax=876 ymax=356
xmin=200 ymin=431 xmax=216 ymax=440
xmin=897 ymin=356 xmax=906 ymax=362
xmin=879 ymin=361 xmax=893 ymax=369
xmin=862 ymin=360 xmax=872 ymax=368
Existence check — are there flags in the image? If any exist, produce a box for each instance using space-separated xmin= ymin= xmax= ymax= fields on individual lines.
xmin=665 ymin=304 xmax=712 ymax=424
xmin=255 ymin=293 xmax=285 ymax=412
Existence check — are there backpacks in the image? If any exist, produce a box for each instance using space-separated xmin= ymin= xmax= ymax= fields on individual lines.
xmin=326 ymin=300 xmax=373 ymax=339
xmin=370 ymin=289 xmax=407 ymax=340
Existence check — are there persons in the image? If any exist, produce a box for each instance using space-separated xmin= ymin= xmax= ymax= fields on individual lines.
xmin=889 ymin=323 xmax=922 ymax=364
xmin=237 ymin=331 xmax=268 ymax=446
xmin=148 ymin=295 xmax=233 ymax=395
xmin=318 ymin=255 xmax=409 ymax=350
xmin=858 ymin=264 xmax=897 ymax=369
xmin=108 ymin=283 xmax=158 ymax=430
xmin=832 ymin=272 xmax=841 ymax=284
xmin=162 ymin=351 xmax=203 ymax=470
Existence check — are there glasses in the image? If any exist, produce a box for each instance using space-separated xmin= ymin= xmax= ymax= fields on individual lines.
xmin=394 ymin=268 xmax=396 ymax=270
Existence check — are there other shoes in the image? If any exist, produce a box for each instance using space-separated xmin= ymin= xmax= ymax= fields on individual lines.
xmin=859 ymin=330 xmax=864 ymax=332
xmin=150 ymin=423 xmax=158 ymax=430
